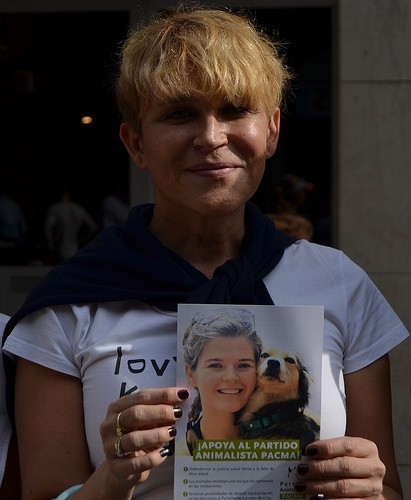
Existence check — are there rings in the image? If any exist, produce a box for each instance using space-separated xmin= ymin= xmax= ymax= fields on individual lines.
xmin=115 ymin=437 xmax=129 ymax=458
xmin=116 ymin=412 xmax=124 ymax=436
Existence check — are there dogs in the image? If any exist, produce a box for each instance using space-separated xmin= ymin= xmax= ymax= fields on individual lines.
xmin=239 ymin=347 xmax=318 ymax=456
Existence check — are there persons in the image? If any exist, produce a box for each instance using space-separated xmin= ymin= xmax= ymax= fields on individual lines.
xmin=0 ymin=173 xmax=315 ymax=265
xmin=175 ymin=308 xmax=318 ymax=458
xmin=0 ymin=10 xmax=407 ymax=500
